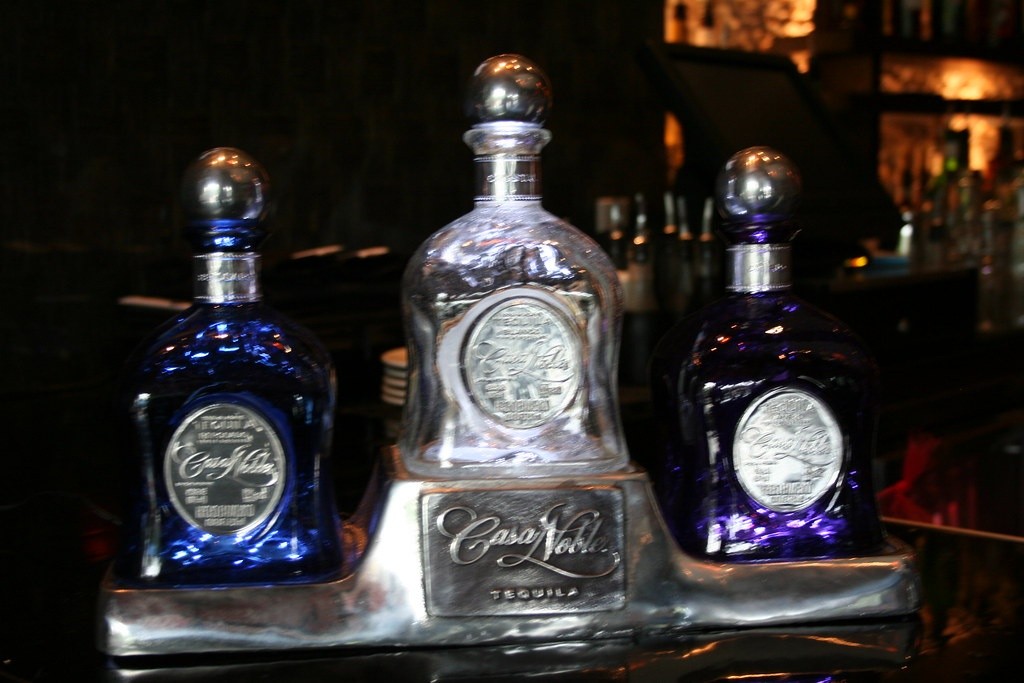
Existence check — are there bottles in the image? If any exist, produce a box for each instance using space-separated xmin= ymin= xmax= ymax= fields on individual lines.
xmin=601 ymin=187 xmax=716 ymax=312
xmin=121 ymin=147 xmax=346 ymax=584
xmin=654 ymin=149 xmax=891 ymax=563
xmin=396 ymin=55 xmax=632 ymax=479
xmin=896 ymin=126 xmax=1024 ymax=326
